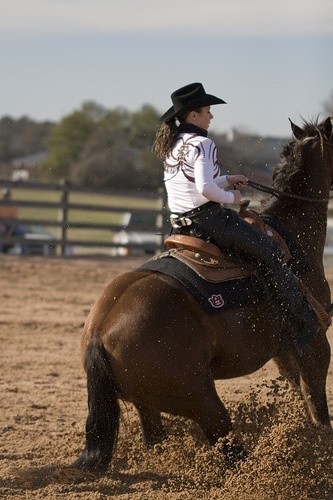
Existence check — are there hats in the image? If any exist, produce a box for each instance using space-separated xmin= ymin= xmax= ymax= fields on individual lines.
xmin=159 ymin=82 xmax=226 ymax=124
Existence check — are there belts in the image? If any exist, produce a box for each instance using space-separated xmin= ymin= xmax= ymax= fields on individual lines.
xmin=171 ymin=200 xmax=217 ymax=219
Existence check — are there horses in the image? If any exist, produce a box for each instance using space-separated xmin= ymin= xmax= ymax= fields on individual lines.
xmin=81 ymin=112 xmax=333 ymax=475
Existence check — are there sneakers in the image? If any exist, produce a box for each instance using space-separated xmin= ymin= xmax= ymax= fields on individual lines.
xmin=289 ymin=302 xmax=331 ymax=334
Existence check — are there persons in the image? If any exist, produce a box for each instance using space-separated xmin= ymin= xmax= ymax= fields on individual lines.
xmin=152 ymin=81 xmax=333 ymax=349
